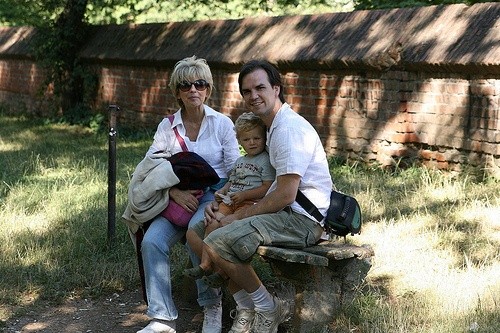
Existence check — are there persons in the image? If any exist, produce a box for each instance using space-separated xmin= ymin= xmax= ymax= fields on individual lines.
xmin=135 ymin=55 xmax=241 ymax=333
xmin=185 ymin=58 xmax=333 ymax=333
xmin=183 ymin=112 xmax=276 ymax=288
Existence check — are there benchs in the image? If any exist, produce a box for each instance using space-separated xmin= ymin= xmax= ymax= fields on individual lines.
xmin=181 ymin=234 xmax=372 ymax=333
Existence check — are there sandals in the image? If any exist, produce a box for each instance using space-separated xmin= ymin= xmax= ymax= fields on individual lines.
xmin=201 ymin=272 xmax=229 ymax=288
xmin=182 ymin=265 xmax=213 ymax=279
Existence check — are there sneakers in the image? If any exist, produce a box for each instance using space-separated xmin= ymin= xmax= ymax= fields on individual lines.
xmin=202 ymin=293 xmax=222 ymax=333
xmin=227 ymin=296 xmax=290 ymax=333
xmin=136 ymin=318 xmax=176 ymax=333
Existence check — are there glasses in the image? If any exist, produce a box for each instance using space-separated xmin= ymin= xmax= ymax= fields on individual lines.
xmin=178 ymin=79 xmax=211 ymax=91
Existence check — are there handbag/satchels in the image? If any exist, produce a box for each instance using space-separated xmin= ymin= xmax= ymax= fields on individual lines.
xmin=325 ymin=191 xmax=361 ymax=236
xmin=159 ymin=189 xmax=203 ymax=228
